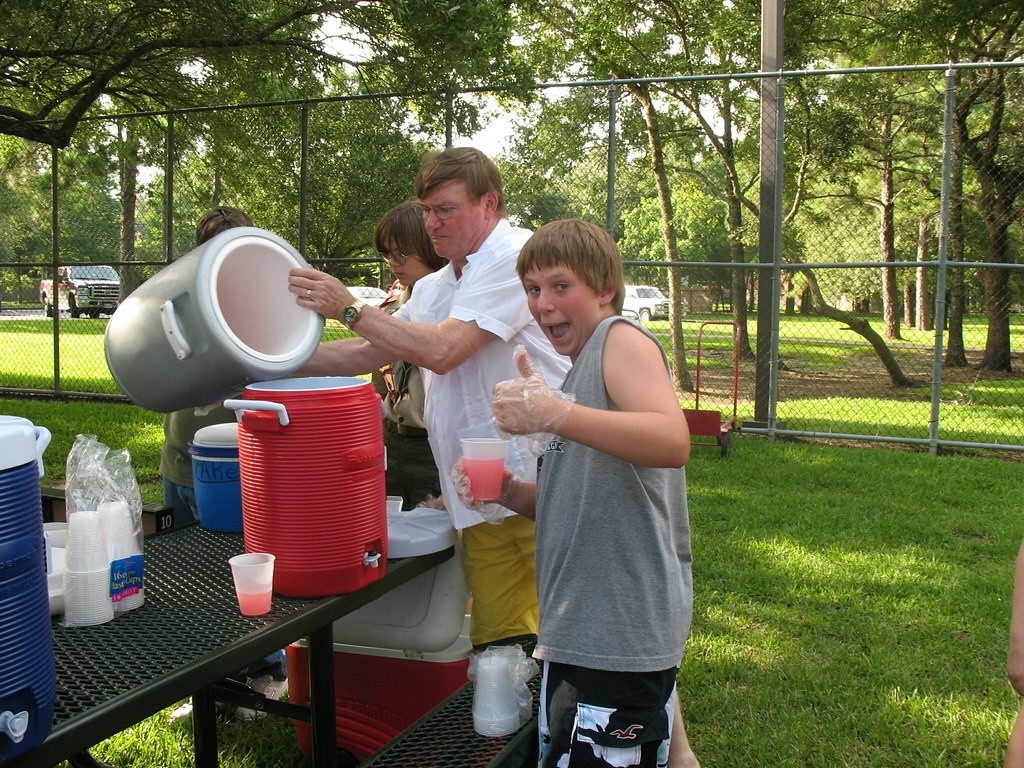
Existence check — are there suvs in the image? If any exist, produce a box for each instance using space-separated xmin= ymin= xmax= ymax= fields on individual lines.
xmin=622 ymin=284 xmax=687 ymax=321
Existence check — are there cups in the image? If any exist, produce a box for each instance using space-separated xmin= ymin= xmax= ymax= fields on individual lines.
xmin=64 ymin=501 xmax=145 ymax=626
xmin=472 ymin=656 xmax=520 ymax=736
xmin=229 ymin=553 xmax=276 ymax=616
xmin=460 ymin=438 xmax=508 ymax=501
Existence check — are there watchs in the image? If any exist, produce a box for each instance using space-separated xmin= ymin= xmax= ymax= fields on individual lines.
xmin=343 ymin=300 xmax=367 ymax=329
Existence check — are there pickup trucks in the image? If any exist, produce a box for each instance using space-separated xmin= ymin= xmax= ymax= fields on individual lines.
xmin=38 ymin=264 xmax=122 ymax=319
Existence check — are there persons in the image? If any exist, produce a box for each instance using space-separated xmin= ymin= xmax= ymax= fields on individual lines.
xmin=158 ymin=147 xmax=702 ymax=768
xmin=449 ymin=219 xmax=690 ymax=768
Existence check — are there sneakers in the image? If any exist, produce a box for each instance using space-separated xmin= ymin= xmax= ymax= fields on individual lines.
xmin=233 ymin=675 xmax=289 ymax=720
xmin=169 ymin=696 xmax=224 ymax=721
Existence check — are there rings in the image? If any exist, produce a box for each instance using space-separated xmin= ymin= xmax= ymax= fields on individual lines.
xmin=306 ymin=290 xmax=311 ymax=298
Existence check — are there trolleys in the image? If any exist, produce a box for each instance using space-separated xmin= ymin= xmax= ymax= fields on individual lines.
xmin=681 ymin=322 xmax=744 ymax=458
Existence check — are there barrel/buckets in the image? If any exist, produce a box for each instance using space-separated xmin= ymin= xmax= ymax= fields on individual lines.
xmin=187 ymin=423 xmax=244 ymax=533
xmin=104 ymin=226 xmax=326 ymax=413
xmin=0 ymin=416 xmax=56 ymax=765
xmin=224 ymin=376 xmax=387 ymax=598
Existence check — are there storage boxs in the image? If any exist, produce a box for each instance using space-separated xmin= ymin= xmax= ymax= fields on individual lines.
xmin=282 ymin=537 xmax=511 ymax=764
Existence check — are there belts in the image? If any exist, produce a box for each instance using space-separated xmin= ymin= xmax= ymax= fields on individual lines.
xmin=384 ymin=418 xmax=428 ymax=436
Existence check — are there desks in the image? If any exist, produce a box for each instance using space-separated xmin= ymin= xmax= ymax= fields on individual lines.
xmin=0 ymin=509 xmax=457 ymax=768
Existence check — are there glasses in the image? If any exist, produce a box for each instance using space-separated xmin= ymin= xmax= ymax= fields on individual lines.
xmin=414 ymin=195 xmax=480 ymax=220
xmin=382 ymin=249 xmax=415 ymax=265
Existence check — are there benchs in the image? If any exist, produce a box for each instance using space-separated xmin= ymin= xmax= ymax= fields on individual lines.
xmin=359 ymin=650 xmax=554 ymax=768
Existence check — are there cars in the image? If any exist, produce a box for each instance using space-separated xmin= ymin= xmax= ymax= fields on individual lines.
xmin=347 ymin=287 xmax=389 ymax=309
